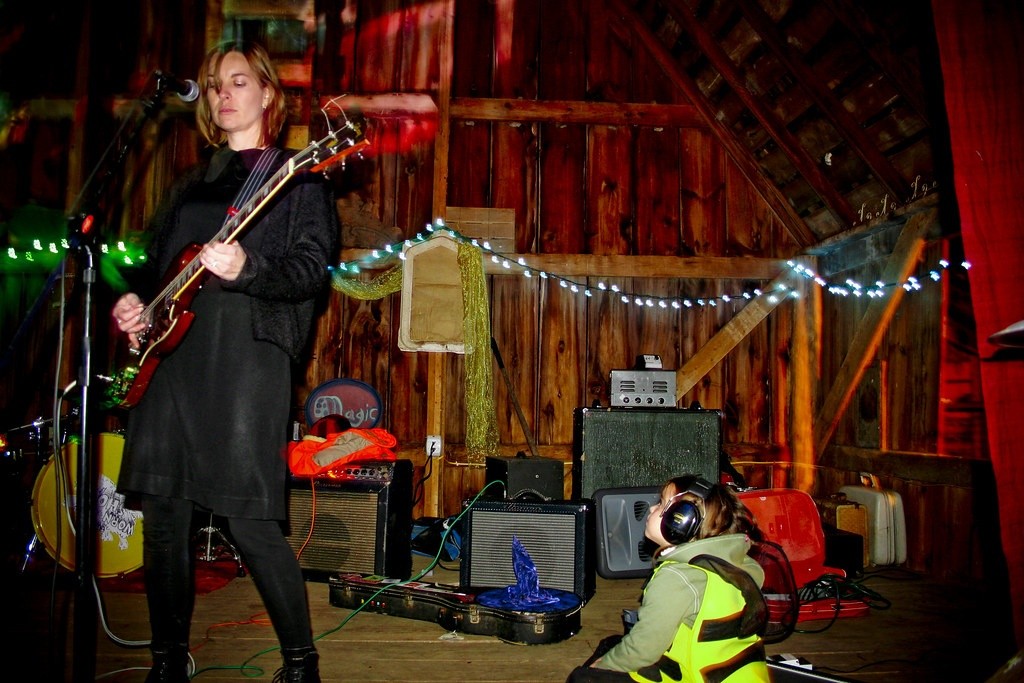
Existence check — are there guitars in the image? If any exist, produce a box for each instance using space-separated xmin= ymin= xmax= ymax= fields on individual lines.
xmin=110 ymin=115 xmax=373 ymax=411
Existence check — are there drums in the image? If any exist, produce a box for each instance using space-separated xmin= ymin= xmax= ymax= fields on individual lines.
xmin=29 ymin=428 xmax=144 ymax=580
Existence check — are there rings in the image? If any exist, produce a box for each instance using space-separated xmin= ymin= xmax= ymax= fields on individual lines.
xmin=117 ymin=319 xmax=122 ymax=323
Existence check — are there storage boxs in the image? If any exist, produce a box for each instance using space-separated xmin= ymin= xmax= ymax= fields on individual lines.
xmin=738 ymin=488 xmax=874 ymax=622
xmin=446 ymin=207 xmax=515 ymax=253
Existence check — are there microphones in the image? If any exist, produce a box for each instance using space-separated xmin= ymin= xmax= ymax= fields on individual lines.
xmin=154 ymin=68 xmax=199 ymax=102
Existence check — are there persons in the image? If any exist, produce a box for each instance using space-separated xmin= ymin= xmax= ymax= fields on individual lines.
xmin=565 ymin=474 xmax=769 ymax=683
xmin=111 ymin=44 xmax=343 ymax=681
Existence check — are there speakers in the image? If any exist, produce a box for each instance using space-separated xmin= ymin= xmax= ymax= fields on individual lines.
xmin=460 ymin=406 xmax=725 ymax=607
xmin=283 ymin=458 xmax=414 ymax=584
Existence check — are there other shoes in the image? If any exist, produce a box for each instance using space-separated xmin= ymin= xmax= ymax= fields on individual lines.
xmin=143 ymin=646 xmax=191 ymax=683
xmin=271 ymin=646 xmax=321 ymax=683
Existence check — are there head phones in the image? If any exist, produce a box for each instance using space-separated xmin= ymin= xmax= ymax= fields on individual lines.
xmin=660 ymin=478 xmax=713 ymax=545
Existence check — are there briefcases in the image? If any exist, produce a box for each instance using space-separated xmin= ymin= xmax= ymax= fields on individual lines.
xmin=812 ymin=492 xmax=873 ymax=568
xmin=838 ymin=470 xmax=907 ymax=569
